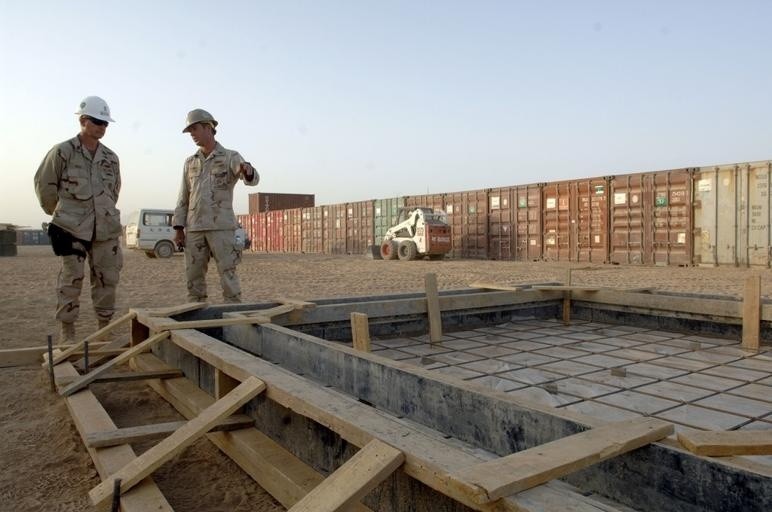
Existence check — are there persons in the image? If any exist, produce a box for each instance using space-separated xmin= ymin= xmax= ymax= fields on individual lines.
xmin=34 ymin=96 xmax=123 ymax=347
xmin=172 ymin=108 xmax=260 ymax=303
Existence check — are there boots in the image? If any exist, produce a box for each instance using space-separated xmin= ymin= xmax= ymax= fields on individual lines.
xmin=59 ymin=321 xmax=76 ymax=344
xmin=98 ymin=319 xmax=123 ymax=341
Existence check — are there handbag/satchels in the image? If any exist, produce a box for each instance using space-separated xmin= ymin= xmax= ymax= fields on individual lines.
xmin=47 ymin=223 xmax=73 ymax=257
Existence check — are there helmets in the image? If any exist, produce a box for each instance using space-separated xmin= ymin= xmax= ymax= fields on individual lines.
xmin=182 ymin=109 xmax=218 ymax=133
xmin=74 ymin=95 xmax=115 ymax=122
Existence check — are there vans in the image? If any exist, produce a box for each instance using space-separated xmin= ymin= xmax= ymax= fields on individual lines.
xmin=126 ymin=208 xmax=251 ymax=258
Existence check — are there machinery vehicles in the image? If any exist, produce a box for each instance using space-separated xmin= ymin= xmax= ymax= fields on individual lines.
xmin=364 ymin=206 xmax=452 ymax=260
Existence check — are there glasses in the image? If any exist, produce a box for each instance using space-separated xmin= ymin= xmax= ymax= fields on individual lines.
xmin=82 ymin=116 xmax=110 ymax=128
